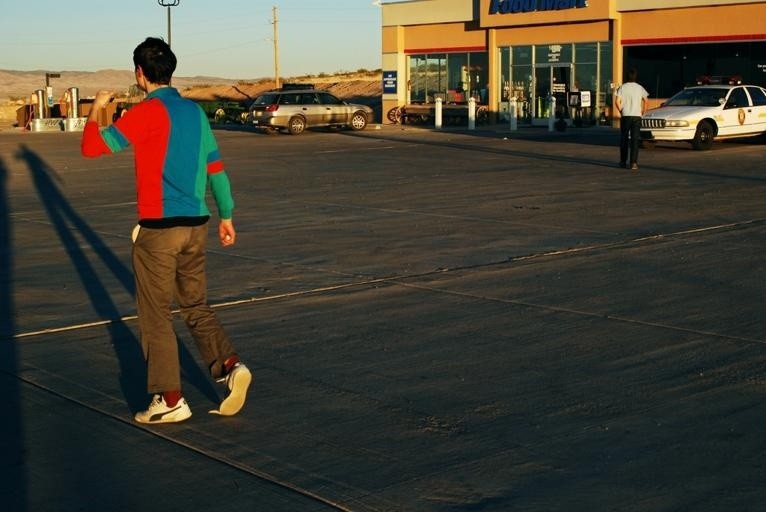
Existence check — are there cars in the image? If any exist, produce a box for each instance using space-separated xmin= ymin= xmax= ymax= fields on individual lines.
xmin=637 ymin=74 xmax=766 ymax=151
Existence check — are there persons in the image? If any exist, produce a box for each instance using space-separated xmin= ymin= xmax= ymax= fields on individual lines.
xmin=456 ymin=82 xmax=465 ymax=93
xmin=81 ymin=38 xmax=252 ymax=424
xmin=614 ymin=68 xmax=650 ymax=170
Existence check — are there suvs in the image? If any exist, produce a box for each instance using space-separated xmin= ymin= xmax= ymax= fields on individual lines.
xmin=244 ymin=89 xmax=376 ymax=136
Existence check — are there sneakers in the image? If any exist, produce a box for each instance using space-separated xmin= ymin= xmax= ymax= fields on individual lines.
xmin=618 ymin=159 xmax=640 ymax=170
xmin=208 ymin=361 xmax=252 ymax=416
xmin=134 ymin=394 xmax=192 ymax=424
